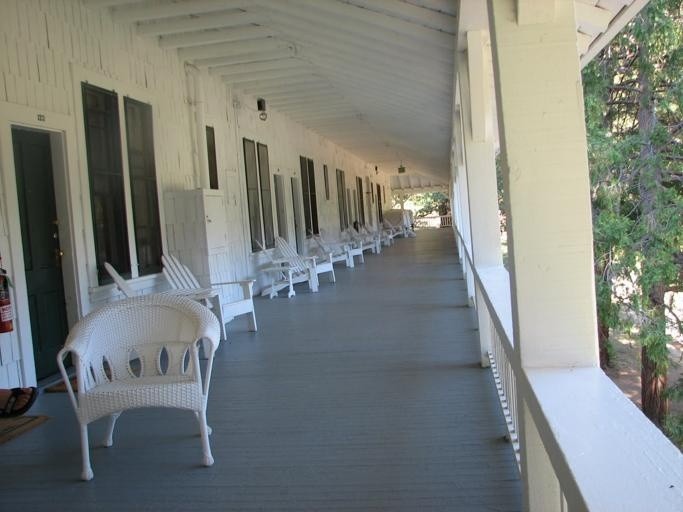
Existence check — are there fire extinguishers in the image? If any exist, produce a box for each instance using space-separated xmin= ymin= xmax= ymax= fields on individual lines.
xmin=0 ymin=268 xmax=14 ymax=333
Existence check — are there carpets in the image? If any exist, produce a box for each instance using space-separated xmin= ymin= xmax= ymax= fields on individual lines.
xmin=43 ymin=367 xmax=138 ymax=393
xmin=0 ymin=413 xmax=49 ymax=445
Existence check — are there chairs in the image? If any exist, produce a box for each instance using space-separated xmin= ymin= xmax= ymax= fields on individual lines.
xmin=105 ymin=259 xmax=218 ymax=364
xmin=254 ymin=218 xmax=415 ymax=299
xmin=159 ymin=247 xmax=260 ymax=342
xmin=58 ymin=292 xmax=224 ymax=480
